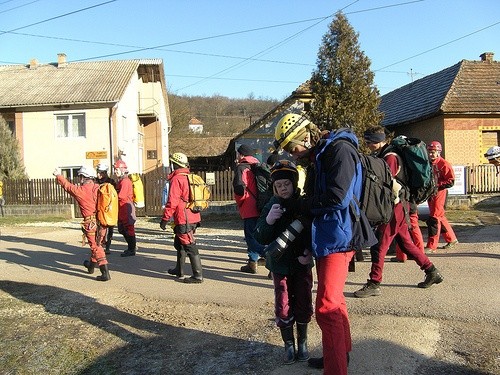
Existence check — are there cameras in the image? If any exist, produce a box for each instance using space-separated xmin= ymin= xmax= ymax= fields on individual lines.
xmin=260 ymin=207 xmax=313 ymax=263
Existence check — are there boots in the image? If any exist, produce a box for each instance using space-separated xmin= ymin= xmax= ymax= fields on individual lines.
xmin=241 ymin=261 xmax=258 ymax=274
xmin=168 ymin=251 xmax=204 ymax=283
xmin=105 ymin=238 xmax=111 ymax=254
xmin=97 ymin=264 xmax=110 ymax=281
xmin=280 ymin=322 xmax=310 ymax=364
xmin=84 ymin=259 xmax=98 ymax=274
xmin=120 ymin=241 xmax=136 ymax=257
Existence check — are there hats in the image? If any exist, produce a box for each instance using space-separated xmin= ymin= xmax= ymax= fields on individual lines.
xmin=238 ymin=144 xmax=254 ymax=156
xmin=267 ymin=150 xmax=280 ymax=164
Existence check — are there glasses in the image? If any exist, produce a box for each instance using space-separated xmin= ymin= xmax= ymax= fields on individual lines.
xmin=363 ymin=141 xmax=373 ymax=145
xmin=270 ymin=161 xmax=299 ymax=173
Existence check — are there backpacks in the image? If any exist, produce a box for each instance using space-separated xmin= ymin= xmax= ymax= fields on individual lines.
xmin=329 ymin=132 xmax=435 ymax=228
xmin=238 ymin=162 xmax=272 ymax=209
xmin=179 ymin=172 xmax=212 ymax=213
xmin=129 ymin=172 xmax=145 ymax=208
xmin=97 ymin=182 xmax=119 ymax=227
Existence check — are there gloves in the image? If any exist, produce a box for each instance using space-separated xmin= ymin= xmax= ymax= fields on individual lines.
xmin=160 ymin=220 xmax=168 ymax=230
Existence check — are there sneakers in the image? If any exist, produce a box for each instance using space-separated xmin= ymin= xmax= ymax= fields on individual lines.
xmin=418 ymin=268 xmax=444 ymax=288
xmin=354 ymin=278 xmax=382 ymax=298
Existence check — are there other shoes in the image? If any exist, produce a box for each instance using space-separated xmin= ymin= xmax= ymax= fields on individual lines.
xmin=308 ymin=351 xmax=350 ymax=368
xmin=424 ymin=240 xmax=458 ymax=254
xmin=391 ymin=257 xmax=404 ymax=262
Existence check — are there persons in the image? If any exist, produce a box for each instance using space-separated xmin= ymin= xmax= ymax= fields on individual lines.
xmin=160 ymin=153 xmax=203 ymax=283
xmin=94 ymin=164 xmax=117 ymax=254
xmin=352 ymin=128 xmax=443 ymax=298
xmin=231 ymin=144 xmax=308 ymax=283
xmin=390 ymin=141 xmax=458 ymax=263
xmin=275 ymin=113 xmax=364 ymax=375
xmin=484 ymin=146 xmax=500 ymax=166
xmin=114 ymin=160 xmax=137 ymax=256
xmin=53 ymin=164 xmax=111 ymax=281
xmin=254 ymin=161 xmax=314 ymax=364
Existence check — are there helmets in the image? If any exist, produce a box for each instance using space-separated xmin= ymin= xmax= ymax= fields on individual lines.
xmin=271 ymin=160 xmax=299 ymax=180
xmin=274 ymin=113 xmax=311 ymax=149
xmin=77 ymin=159 xmax=128 ymax=178
xmin=484 ymin=146 xmax=500 ymax=160
xmin=428 ymin=141 xmax=442 ymax=151
xmin=169 ymin=152 xmax=188 ymax=168
